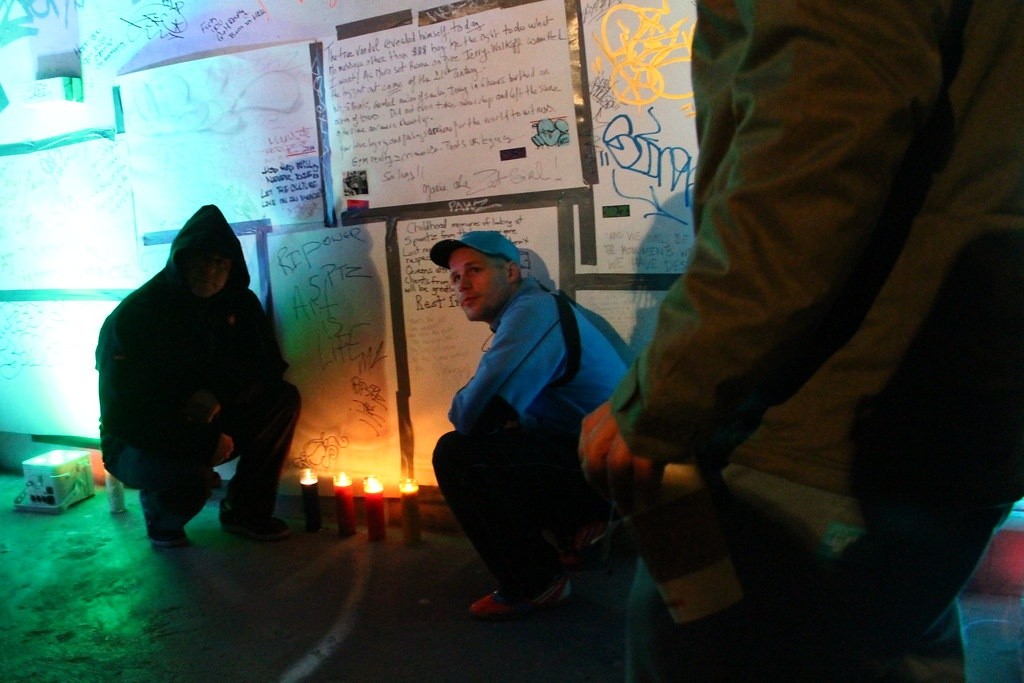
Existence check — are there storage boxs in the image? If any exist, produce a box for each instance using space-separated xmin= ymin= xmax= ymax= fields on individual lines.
xmin=23 ymin=450 xmax=96 ymax=513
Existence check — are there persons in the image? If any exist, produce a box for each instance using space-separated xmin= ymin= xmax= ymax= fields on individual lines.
xmin=95 ymin=204 xmax=302 ymax=545
xmin=578 ymin=0 xmax=1024 ymax=683
xmin=429 ymin=231 xmax=638 ymax=619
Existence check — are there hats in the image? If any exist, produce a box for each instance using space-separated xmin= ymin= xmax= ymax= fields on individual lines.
xmin=429 ymin=231 xmax=520 ymax=270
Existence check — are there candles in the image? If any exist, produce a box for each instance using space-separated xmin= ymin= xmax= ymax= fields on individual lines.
xmin=363 ymin=475 xmax=387 ymax=543
xmin=399 ymin=477 xmax=421 ymax=536
xmin=333 ymin=471 xmax=357 ymax=539
xmin=298 ymin=466 xmax=323 ymax=534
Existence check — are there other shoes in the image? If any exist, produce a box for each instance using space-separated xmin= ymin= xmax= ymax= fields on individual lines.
xmin=468 ymin=575 xmax=584 ymax=620
xmin=560 ymin=517 xmax=611 ymax=560
xmin=219 ymin=499 xmax=290 ymax=540
xmin=145 ymin=514 xmax=187 ymax=547
